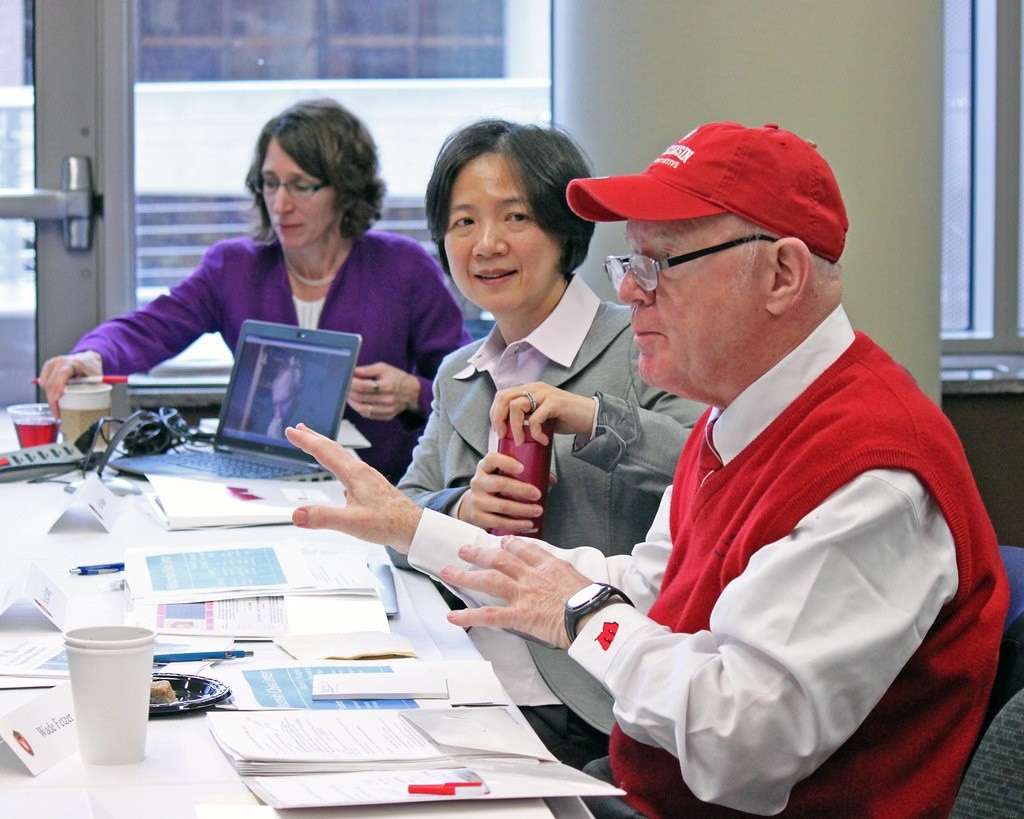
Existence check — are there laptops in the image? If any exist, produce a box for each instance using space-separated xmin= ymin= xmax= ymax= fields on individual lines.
xmin=109 ymin=318 xmax=363 ymax=481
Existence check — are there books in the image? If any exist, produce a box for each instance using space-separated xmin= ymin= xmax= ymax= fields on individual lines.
xmin=0 ymin=464 xmax=627 ymax=809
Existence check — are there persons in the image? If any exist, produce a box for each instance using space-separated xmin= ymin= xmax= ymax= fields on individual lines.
xmin=283 ymin=121 xmax=1012 ymax=819
xmin=40 ymin=100 xmax=473 ymax=487
xmin=251 ymin=357 xmax=316 ymax=439
xmin=383 ymin=119 xmax=711 ymax=569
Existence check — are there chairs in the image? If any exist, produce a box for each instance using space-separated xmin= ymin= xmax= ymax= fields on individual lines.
xmin=946 ymin=539 xmax=1024 ymax=816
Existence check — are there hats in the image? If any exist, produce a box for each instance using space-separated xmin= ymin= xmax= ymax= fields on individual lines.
xmin=566 ymin=122 xmax=850 ymax=263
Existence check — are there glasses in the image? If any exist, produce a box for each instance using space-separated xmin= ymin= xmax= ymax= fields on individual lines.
xmin=253 ymin=173 xmax=330 ymax=199
xmin=604 ymin=234 xmax=779 ymax=292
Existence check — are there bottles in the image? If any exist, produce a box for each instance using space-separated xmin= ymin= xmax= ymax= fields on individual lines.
xmin=491 ymin=416 xmax=554 ymax=540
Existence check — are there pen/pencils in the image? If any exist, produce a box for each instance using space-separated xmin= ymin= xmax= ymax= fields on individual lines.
xmin=155 ymin=650 xmax=254 ymax=663
xmin=70 ymin=563 xmax=124 ymax=575
xmin=28 ymin=375 xmax=127 ymax=384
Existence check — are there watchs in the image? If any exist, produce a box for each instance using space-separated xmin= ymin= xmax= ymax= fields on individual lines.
xmin=565 ymin=583 xmax=636 ymax=643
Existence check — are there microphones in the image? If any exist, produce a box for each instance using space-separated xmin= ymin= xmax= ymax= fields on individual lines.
xmin=96 ymin=410 xmax=147 ymax=476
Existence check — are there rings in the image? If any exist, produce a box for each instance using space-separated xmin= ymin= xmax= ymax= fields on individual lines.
xmin=524 ymin=392 xmax=537 ymax=413
xmin=373 ymin=381 xmax=380 ymax=394
xmin=67 ymin=363 xmax=77 ymax=376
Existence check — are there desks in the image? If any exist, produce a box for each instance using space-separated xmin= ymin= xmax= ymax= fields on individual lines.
xmin=0 ymin=415 xmax=557 ymax=819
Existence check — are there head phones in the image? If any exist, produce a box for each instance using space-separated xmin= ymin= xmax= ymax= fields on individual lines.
xmin=121 ymin=407 xmax=190 ymax=457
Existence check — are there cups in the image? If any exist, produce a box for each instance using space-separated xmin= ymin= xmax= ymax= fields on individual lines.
xmin=58 ymin=384 xmax=113 ymax=453
xmin=63 ymin=625 xmax=157 ymax=765
xmin=6 ymin=403 xmax=61 ymax=448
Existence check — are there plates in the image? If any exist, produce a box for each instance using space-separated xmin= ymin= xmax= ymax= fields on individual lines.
xmin=149 ymin=672 xmax=233 ymax=713
